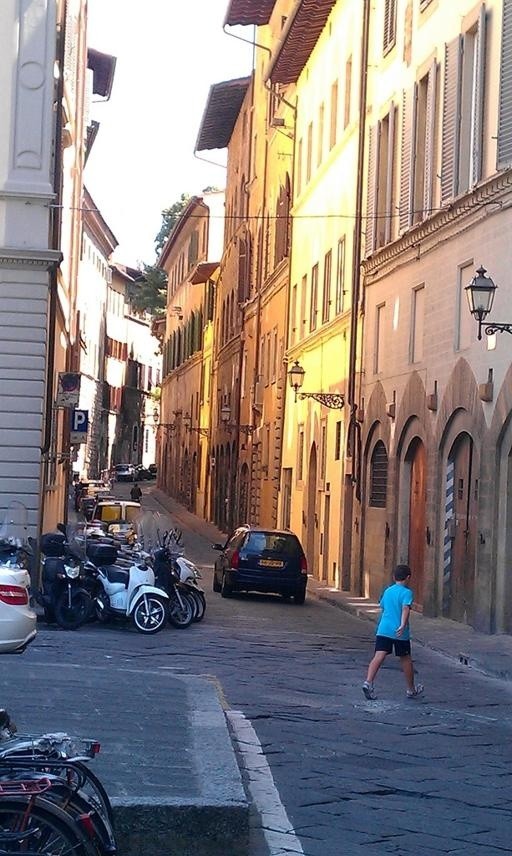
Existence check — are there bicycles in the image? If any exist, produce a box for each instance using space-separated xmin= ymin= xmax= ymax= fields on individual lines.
xmin=0 ymin=708 xmax=119 ymax=856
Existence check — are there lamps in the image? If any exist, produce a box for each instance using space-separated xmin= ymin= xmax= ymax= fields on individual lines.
xmin=288 ymin=360 xmax=344 ymax=408
xmin=464 ymin=263 xmax=511 ymax=341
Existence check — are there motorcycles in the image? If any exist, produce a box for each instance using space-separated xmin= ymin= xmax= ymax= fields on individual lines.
xmin=38 ymin=521 xmax=208 ymax=633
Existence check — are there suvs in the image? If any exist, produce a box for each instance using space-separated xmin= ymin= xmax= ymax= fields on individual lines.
xmin=211 ymin=523 xmax=308 ymax=605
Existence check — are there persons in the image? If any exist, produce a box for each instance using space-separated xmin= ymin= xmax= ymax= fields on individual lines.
xmin=129 ymin=482 xmax=142 ymax=500
xmin=360 ymin=564 xmax=425 ymax=701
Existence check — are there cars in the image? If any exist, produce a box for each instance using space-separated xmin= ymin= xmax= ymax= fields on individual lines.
xmin=110 ymin=461 xmax=157 ymax=482
xmin=73 ymin=478 xmax=142 ymax=537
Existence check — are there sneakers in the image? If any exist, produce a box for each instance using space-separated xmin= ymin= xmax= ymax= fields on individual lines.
xmin=362 ymin=681 xmax=377 ymax=700
xmin=406 ymin=684 xmax=423 ymax=698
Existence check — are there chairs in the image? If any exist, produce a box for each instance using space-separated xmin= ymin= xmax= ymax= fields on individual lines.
xmin=274 ymin=540 xmax=285 ymax=549
